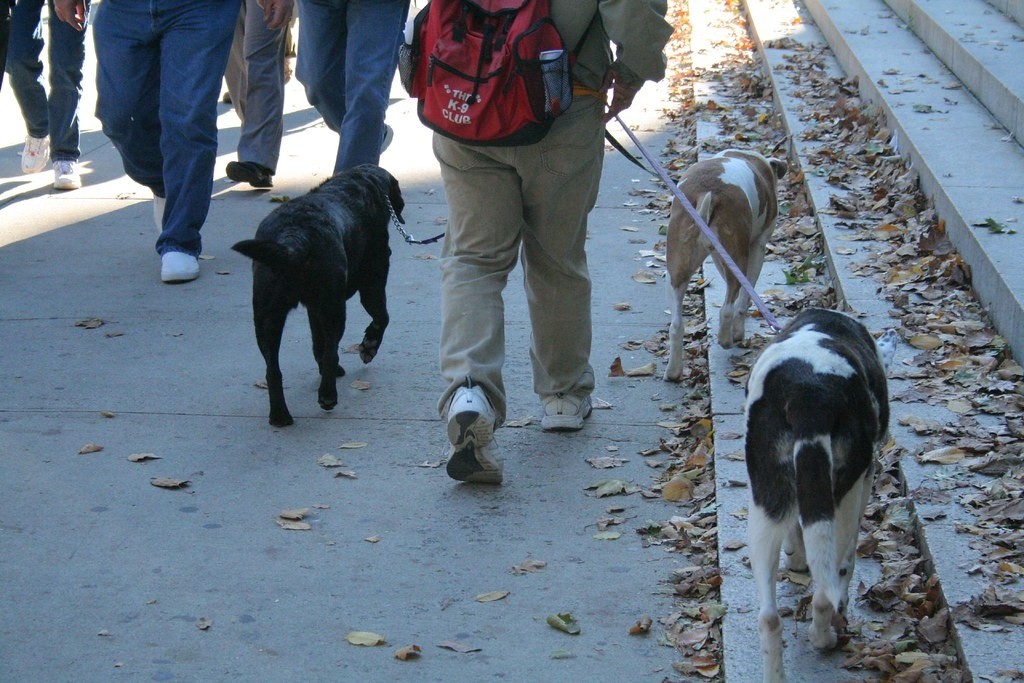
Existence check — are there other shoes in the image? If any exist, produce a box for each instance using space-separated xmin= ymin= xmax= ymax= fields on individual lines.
xmin=162 ymin=251 xmax=200 ymax=282
xmin=153 ymin=194 xmax=166 ymax=233
xmin=380 ymin=124 xmax=394 ymax=152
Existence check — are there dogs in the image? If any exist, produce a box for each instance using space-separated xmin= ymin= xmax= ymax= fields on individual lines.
xmin=229 ymin=162 xmax=405 ymax=427
xmin=743 ymin=306 xmax=891 ymax=683
xmin=663 ymin=148 xmax=788 ymax=384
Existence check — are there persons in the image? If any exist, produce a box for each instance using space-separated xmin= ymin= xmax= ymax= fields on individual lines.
xmin=52 ymin=0 xmax=295 ymax=283
xmin=295 ymin=0 xmax=410 ymax=178
xmin=223 ymin=0 xmax=290 ymax=187
xmin=431 ymin=1 xmax=676 ymax=485
xmin=0 ymin=0 xmax=92 ymax=190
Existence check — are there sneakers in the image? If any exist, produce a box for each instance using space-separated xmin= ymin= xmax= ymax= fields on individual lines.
xmin=539 ymin=391 xmax=592 ymax=430
xmin=446 ymin=375 xmax=504 ymax=484
xmin=227 ymin=160 xmax=274 ymax=188
xmin=21 ymin=135 xmax=50 ymax=173
xmin=53 ymin=160 xmax=81 ymax=189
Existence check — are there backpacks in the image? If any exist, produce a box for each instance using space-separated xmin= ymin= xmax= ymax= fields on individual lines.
xmin=397 ymin=0 xmax=599 ymax=146
